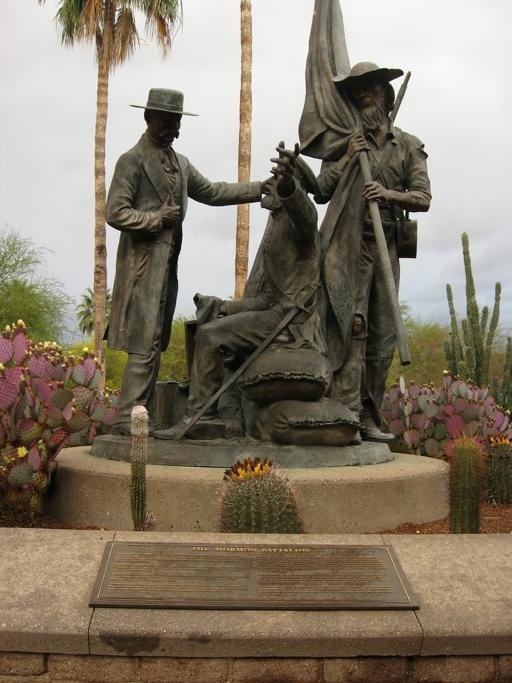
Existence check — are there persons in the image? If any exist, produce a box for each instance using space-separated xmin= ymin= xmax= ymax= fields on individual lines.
xmin=101 ymin=86 xmax=264 ymax=438
xmin=154 ymin=142 xmax=320 ymax=442
xmin=310 ymin=62 xmax=431 ymax=444
